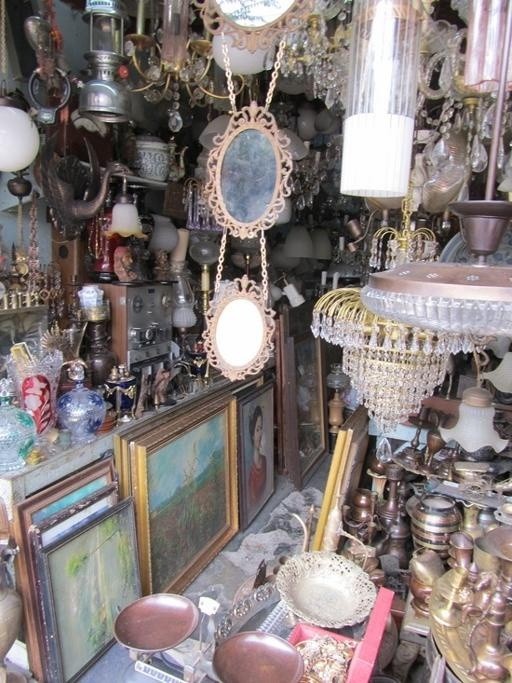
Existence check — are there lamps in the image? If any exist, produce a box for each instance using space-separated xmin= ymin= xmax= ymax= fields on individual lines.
xmin=101 ymin=174 xmax=149 ymax=241
xmin=278 ymin=0 xmax=511 ymax=463
xmin=77 ymin=0 xmax=132 ymax=123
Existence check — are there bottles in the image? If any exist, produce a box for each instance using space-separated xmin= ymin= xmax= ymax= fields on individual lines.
xmin=0 ymin=377 xmax=39 ymax=471
xmin=56 ymin=364 xmax=107 ymax=448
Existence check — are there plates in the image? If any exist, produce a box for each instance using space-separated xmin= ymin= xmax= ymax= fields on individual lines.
xmin=114 ymin=592 xmax=200 ymax=653
xmin=276 ymin=549 xmax=378 ymax=631
xmin=211 ymin=630 xmax=305 ymax=683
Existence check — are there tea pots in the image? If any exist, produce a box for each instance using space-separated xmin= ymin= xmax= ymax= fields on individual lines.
xmin=166 ymin=135 xmax=190 ymax=183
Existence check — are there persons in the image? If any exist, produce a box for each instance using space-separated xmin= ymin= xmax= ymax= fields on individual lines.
xmin=248 ymin=405 xmax=267 ymax=506
xmin=114 ymin=246 xmax=170 ymax=283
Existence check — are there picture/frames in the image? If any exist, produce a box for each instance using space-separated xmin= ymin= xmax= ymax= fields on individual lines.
xmin=10 ymin=369 xmax=278 ymax=683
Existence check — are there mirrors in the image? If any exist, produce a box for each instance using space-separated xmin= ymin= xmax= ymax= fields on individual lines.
xmin=196 ymin=0 xmax=314 ymax=54
xmin=201 ymin=278 xmax=277 ymax=383
xmin=204 ymin=100 xmax=294 ymax=240
